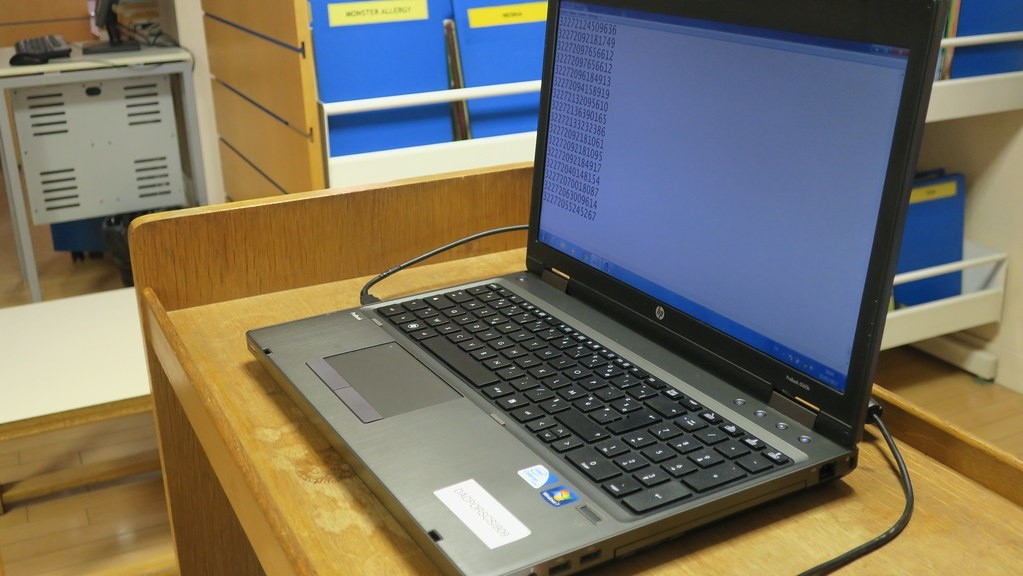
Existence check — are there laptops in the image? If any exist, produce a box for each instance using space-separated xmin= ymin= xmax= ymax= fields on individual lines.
xmin=247 ymin=0 xmax=951 ymax=575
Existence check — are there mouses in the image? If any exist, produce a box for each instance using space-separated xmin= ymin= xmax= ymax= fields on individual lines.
xmin=8 ymin=53 xmax=49 ymax=66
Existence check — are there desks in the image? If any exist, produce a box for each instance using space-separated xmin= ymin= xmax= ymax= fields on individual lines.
xmin=126 ymin=162 xmax=1023 ymax=576
xmin=0 ymin=41 xmax=196 ymax=302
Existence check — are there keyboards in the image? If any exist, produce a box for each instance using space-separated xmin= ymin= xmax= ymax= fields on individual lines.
xmin=15 ymin=35 xmax=71 ymax=59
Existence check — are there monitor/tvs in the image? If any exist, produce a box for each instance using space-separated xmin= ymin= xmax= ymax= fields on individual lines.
xmin=82 ymin=0 xmax=140 ymax=55
